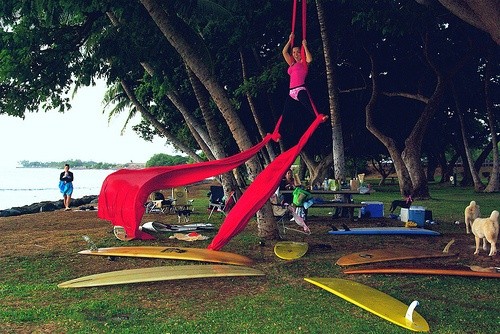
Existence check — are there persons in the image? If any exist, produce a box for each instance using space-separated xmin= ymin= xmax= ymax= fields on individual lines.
xmin=60 ymin=163 xmax=74 ymax=211
xmin=278 ymin=169 xmax=301 ymax=205
xmin=266 ymin=32 xmax=329 ymax=142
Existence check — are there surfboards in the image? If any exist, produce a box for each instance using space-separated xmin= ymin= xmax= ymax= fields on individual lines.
xmin=273 ymin=241 xmax=309 ymax=260
xmin=56 ymin=265 xmax=263 ymax=289
xmin=328 ymin=224 xmax=440 ymax=236
xmin=79 ymin=247 xmax=256 ymax=266
xmin=342 ymin=269 xmax=500 ymax=278
xmin=304 ymin=277 xmax=428 ymax=331
xmin=336 ymin=247 xmax=457 ymax=266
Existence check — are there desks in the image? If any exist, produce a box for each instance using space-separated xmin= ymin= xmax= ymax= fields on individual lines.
xmin=280 ymin=189 xmax=360 ymax=219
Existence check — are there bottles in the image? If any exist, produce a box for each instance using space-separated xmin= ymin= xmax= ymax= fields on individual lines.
xmin=324 ymin=178 xmax=329 ymax=190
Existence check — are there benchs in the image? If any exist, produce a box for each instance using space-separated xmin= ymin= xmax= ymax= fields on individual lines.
xmin=279 ymin=203 xmax=365 ymax=207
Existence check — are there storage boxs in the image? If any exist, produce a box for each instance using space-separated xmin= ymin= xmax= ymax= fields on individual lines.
xmin=409 ymin=210 xmax=432 ymax=226
xmin=360 ymin=201 xmax=384 ymax=218
xmin=400 ymin=208 xmax=425 ymax=222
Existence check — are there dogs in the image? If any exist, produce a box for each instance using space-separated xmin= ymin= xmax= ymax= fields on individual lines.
xmin=390 ymin=199 xmax=414 ymax=215
xmin=464 ymin=199 xmax=480 ymax=234
xmin=472 ymin=209 xmax=500 ymax=256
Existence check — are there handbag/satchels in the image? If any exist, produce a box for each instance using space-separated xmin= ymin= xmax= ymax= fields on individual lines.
xmin=293 ymin=187 xmax=314 ymax=207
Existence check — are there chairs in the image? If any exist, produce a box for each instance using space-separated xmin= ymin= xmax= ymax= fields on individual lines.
xmin=172 ymin=187 xmax=194 ymax=213
xmin=149 ymin=192 xmax=172 ymax=214
xmin=208 ymin=185 xmax=225 ymax=220
